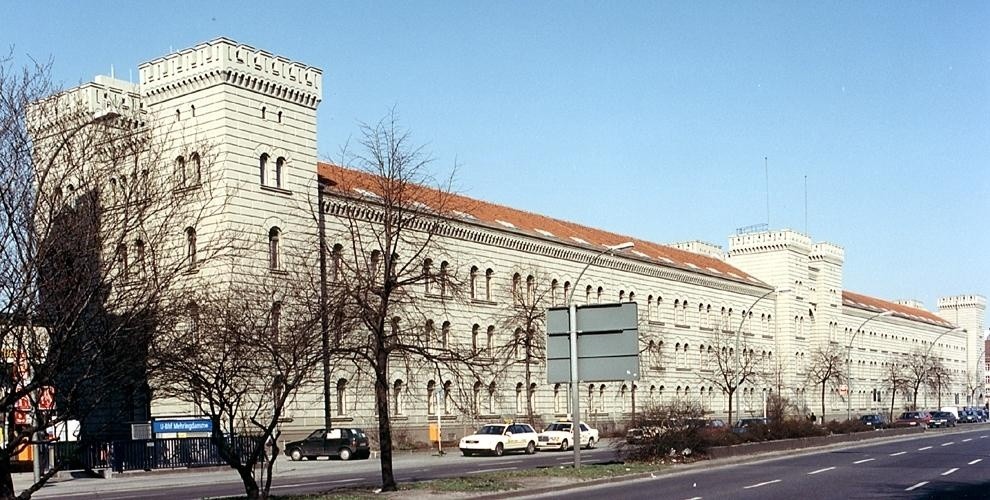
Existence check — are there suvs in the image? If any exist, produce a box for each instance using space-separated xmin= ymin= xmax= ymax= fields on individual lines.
xmin=285 ymin=428 xmax=369 ymax=461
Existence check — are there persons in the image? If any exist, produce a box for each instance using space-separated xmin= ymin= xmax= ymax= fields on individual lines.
xmin=810 ymin=412 xmax=816 ymax=424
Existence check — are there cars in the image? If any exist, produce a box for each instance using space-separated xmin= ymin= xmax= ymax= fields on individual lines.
xmin=894 ymin=411 xmax=957 ymax=428
xmin=861 ymin=415 xmax=883 ymax=427
xmin=626 ymin=417 xmax=765 ymax=444
xmin=958 ymin=409 xmax=986 ymax=423
xmin=458 ymin=418 xmax=599 ymax=457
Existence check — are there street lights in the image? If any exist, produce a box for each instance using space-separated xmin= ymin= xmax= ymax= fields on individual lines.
xmin=28 ymin=111 xmax=122 ymax=484
xmin=736 ymin=286 xmax=791 ymax=425
xmin=566 ymin=241 xmax=634 ymax=421
xmin=924 ymin=326 xmax=964 ymax=409
xmin=847 ymin=312 xmax=895 ymax=422
